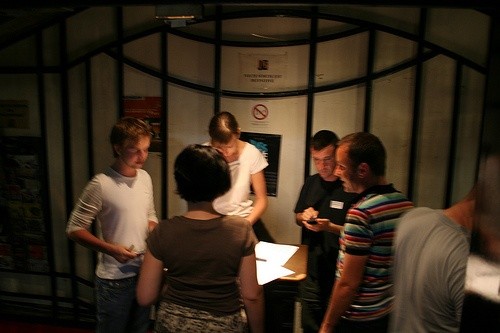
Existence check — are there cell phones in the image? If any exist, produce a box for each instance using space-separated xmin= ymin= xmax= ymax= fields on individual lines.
xmin=307 ymin=220 xmax=318 ymax=225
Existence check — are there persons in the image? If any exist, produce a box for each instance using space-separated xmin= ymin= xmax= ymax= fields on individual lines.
xmin=294 ymin=130 xmax=358 ymax=333
xmin=393 ymin=183 xmax=478 ymax=333
xmin=202 ymin=112 xmax=268 ymax=225
xmin=137 ymin=144 xmax=263 ymax=333
xmin=65 ymin=117 xmax=158 ymax=333
xmin=318 ymin=132 xmax=415 ymax=333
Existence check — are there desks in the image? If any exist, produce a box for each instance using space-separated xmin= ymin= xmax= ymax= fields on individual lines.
xmin=255 ymin=244 xmax=309 ymax=333
